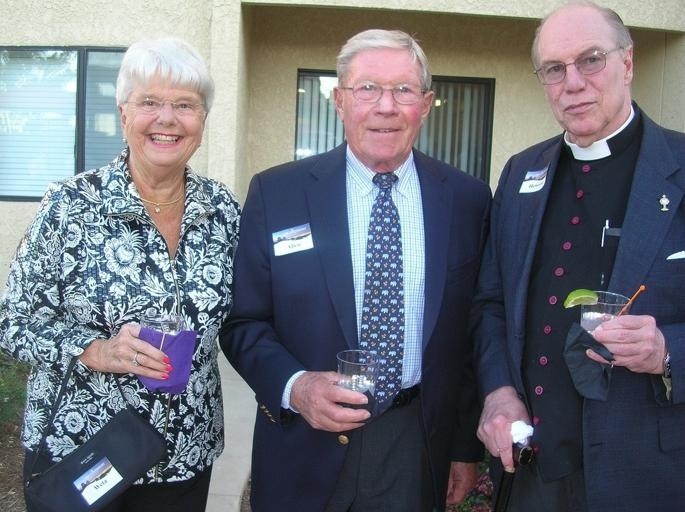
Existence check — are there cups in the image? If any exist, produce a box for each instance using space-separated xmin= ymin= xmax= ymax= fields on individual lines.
xmin=580 ymin=290 xmax=631 ymax=332
xmin=139 ymin=314 xmax=185 ymax=336
xmin=336 ymin=349 xmax=380 ymax=423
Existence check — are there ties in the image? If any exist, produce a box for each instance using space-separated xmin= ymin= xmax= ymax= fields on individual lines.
xmin=359 ymin=171 xmax=405 ymax=420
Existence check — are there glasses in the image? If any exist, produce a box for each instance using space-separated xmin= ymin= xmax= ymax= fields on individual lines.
xmin=123 ymin=96 xmax=207 ymax=116
xmin=336 ymin=80 xmax=430 ymax=105
xmin=532 ymin=47 xmax=623 ymax=86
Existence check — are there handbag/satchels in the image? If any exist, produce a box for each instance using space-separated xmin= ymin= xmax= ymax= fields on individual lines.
xmin=23 ymin=402 xmax=168 ymax=511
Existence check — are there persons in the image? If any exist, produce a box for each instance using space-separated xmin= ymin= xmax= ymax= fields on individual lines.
xmin=468 ymin=1 xmax=685 ymax=512
xmin=0 ymin=27 xmax=245 ymax=511
xmin=219 ymin=25 xmax=493 ymax=512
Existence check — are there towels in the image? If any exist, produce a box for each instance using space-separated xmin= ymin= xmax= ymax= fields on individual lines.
xmin=131 ymin=327 xmax=196 ymax=397
xmin=563 ymin=323 xmax=615 ymax=402
xmin=342 ymin=391 xmax=375 ymax=424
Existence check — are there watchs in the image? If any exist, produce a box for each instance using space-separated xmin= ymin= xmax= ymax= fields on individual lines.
xmin=663 ymin=344 xmax=671 ymax=378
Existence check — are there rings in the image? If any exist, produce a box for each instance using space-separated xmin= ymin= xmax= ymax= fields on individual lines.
xmin=132 ymin=351 xmax=139 ymax=366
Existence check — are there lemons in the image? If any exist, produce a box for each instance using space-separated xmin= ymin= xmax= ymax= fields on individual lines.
xmin=563 ymin=288 xmax=599 ymax=310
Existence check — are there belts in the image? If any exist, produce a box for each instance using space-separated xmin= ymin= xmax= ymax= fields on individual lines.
xmin=392 ymin=385 xmax=418 ymax=407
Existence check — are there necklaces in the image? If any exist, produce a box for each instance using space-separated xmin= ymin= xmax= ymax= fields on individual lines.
xmin=138 ymin=191 xmax=185 ymax=213
